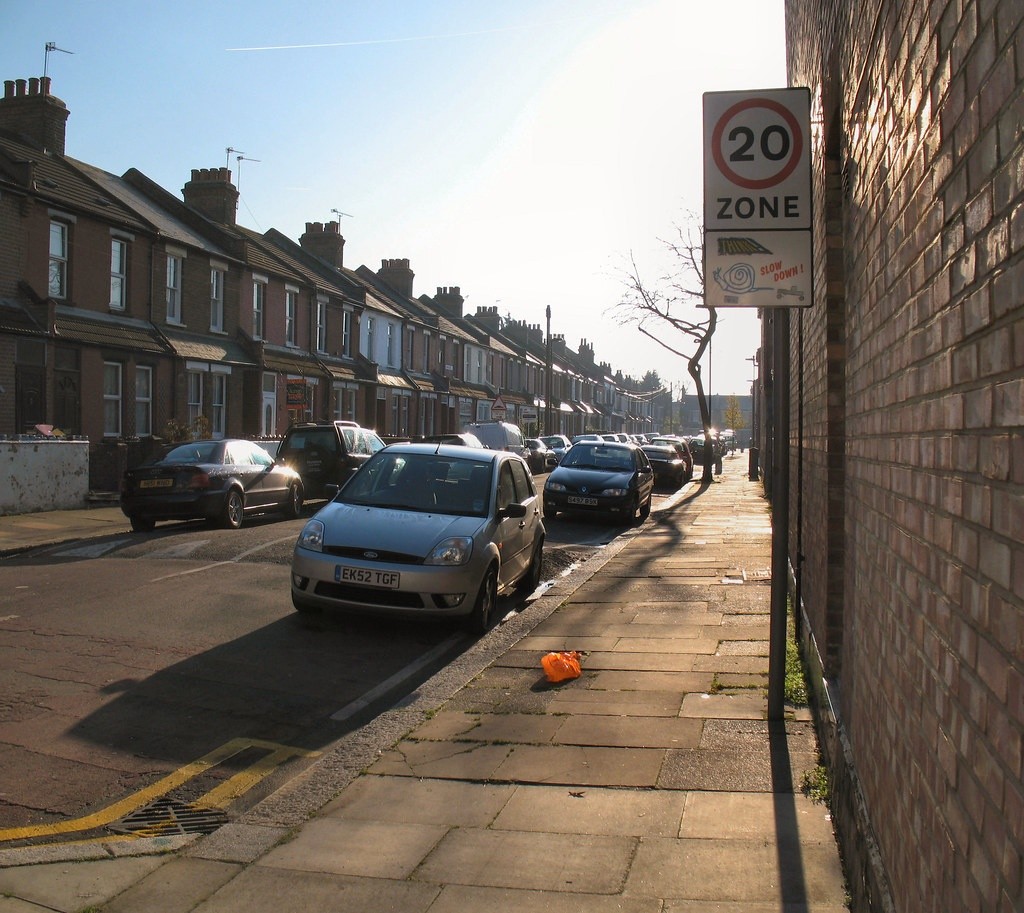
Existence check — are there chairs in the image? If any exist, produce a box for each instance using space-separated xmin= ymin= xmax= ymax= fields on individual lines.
xmin=397 ymin=457 xmax=437 ymax=506
xmin=582 ymin=454 xmax=598 ymax=465
xmin=675 ymin=444 xmax=684 ymax=453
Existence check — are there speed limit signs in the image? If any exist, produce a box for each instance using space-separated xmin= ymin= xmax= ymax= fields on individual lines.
xmin=702 ymin=86 xmax=814 ymax=307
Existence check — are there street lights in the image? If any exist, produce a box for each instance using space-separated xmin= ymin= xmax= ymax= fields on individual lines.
xmin=549 ymin=338 xmax=563 ymax=434
xmin=694 ymin=338 xmax=711 ymax=428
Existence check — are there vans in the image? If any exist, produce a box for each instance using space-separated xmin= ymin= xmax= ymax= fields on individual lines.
xmin=470 ymin=421 xmax=532 ymax=472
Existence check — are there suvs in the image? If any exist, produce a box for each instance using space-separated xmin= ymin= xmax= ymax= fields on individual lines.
xmin=274 ymin=420 xmax=406 ymax=501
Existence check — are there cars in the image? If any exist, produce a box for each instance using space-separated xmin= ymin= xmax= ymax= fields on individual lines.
xmin=651 ymin=429 xmax=736 ymax=478
xmin=543 ymin=439 xmax=654 ymax=521
xmin=290 ymin=441 xmax=546 ymax=629
xmin=422 ymin=434 xmax=489 ymax=449
xmin=638 ymin=445 xmax=686 ymax=487
xmin=525 ymin=432 xmax=659 ymax=472
xmin=119 ymin=438 xmax=303 ymax=531
